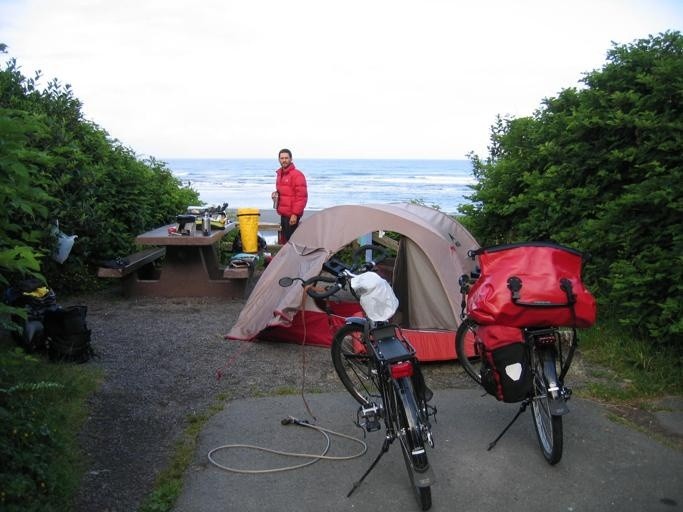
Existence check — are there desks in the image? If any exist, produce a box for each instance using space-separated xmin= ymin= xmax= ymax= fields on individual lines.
xmin=129 ymin=219 xmax=236 ymax=297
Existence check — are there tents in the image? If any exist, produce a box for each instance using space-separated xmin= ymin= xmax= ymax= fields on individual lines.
xmin=223 ymin=201 xmax=482 ymax=362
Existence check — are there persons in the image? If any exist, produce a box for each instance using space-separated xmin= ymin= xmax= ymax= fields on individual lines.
xmin=272 ymin=149 xmax=308 ymax=245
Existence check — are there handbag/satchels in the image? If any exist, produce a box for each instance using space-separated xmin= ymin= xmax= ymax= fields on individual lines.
xmin=466 ymin=243 xmax=597 ymax=403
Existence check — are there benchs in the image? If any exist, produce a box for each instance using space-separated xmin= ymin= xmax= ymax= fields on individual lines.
xmin=98 ymin=247 xmax=165 ymax=279
xmin=223 ymin=249 xmax=258 ymax=279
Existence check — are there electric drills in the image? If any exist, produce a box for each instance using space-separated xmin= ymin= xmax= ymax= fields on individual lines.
xmin=176 ymin=214 xmax=197 ymax=235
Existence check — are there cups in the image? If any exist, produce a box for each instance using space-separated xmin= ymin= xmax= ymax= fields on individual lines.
xmin=167 ymin=226 xmax=177 ymax=235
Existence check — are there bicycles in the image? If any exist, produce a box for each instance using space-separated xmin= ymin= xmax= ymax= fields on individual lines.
xmin=455 ymin=241 xmax=596 ymax=466
xmin=302 ymin=244 xmax=438 ymax=510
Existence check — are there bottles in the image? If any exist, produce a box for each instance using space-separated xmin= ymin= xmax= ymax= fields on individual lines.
xmin=201 ymin=209 xmax=211 ymax=236
xmin=272 ymin=191 xmax=279 ymax=209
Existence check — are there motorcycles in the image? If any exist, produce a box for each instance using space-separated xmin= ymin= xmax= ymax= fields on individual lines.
xmin=0 ymin=261 xmax=64 ymax=352
